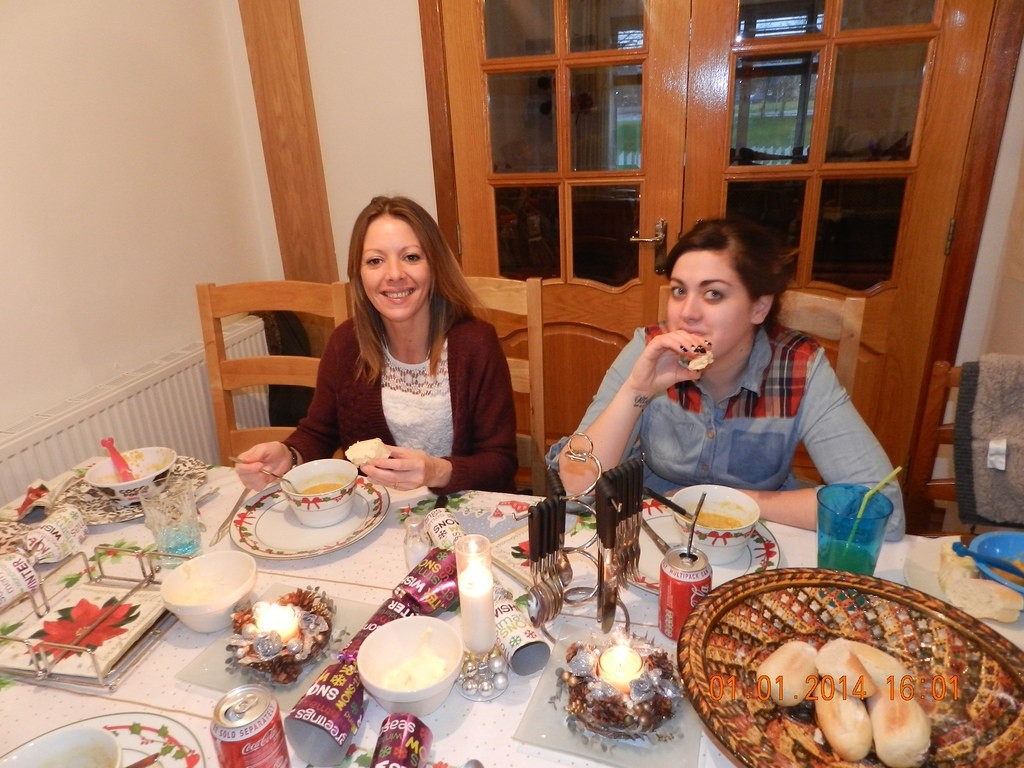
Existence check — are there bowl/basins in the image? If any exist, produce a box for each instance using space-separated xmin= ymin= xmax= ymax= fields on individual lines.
xmin=968 ymin=530 xmax=1024 ymax=593
xmin=84 ymin=446 xmax=177 ymax=506
xmin=0 ymin=727 xmax=122 ymax=768
xmin=160 ymin=550 xmax=257 ymax=632
xmin=357 ymin=616 xmax=464 ymax=717
xmin=671 ymin=485 xmax=761 ymax=564
xmin=280 ymin=459 xmax=358 ymax=528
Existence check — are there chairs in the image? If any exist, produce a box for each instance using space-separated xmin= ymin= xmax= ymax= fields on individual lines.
xmin=193 ymin=283 xmax=348 ymax=464
xmin=900 ymin=362 xmax=981 ymax=543
xmin=658 ymin=284 xmax=866 ymax=482
xmin=460 ymin=273 xmax=547 ymax=496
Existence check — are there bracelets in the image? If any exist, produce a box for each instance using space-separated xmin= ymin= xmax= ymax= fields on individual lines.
xmin=281 ymin=442 xmax=297 ymax=474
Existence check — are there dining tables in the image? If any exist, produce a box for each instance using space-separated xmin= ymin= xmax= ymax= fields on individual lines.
xmin=0 ymin=461 xmax=1024 ymax=768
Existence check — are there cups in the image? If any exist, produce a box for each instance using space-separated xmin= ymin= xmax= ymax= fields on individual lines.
xmin=816 ymin=483 xmax=894 ymax=577
xmin=138 ymin=477 xmax=203 ymax=570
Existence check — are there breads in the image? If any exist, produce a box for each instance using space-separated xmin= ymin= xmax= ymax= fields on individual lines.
xmin=755 ymin=638 xmax=930 ymax=768
xmin=678 ymin=350 xmax=713 ymax=370
xmin=346 ymin=438 xmax=390 ymax=465
xmin=943 ymin=576 xmax=1024 ymax=623
xmin=937 ymin=541 xmax=979 ymax=587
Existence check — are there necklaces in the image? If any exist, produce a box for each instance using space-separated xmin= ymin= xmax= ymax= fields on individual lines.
xmin=382 ymin=334 xmax=430 ymax=361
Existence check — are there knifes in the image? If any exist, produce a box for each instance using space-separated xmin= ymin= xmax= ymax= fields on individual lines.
xmin=209 ymin=486 xmax=250 ymax=546
xmin=639 ymin=513 xmax=674 ymax=555
xmin=595 ymin=457 xmax=644 ymax=634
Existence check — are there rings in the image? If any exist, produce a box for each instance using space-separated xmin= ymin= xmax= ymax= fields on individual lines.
xmin=396 ymin=482 xmax=398 ymax=487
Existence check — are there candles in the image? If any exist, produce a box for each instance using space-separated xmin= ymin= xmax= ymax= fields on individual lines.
xmin=594 ymin=642 xmax=647 ymax=683
xmin=257 ymin=598 xmax=301 ymax=639
xmin=454 ymin=534 xmax=497 ymax=653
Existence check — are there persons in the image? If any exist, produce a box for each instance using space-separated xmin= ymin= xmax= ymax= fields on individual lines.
xmin=234 ymin=193 xmax=518 ymax=494
xmin=542 ymin=217 xmax=907 ymax=543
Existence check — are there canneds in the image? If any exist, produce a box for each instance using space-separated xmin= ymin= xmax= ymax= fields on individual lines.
xmin=209 ymin=684 xmax=292 ymax=768
xmin=659 ymin=545 xmax=713 ymax=641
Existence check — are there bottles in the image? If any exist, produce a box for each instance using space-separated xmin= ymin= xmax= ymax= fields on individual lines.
xmin=404 ymin=517 xmax=431 ymax=573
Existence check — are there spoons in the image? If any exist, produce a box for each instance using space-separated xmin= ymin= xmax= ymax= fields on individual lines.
xmin=527 ymin=495 xmax=573 ymax=629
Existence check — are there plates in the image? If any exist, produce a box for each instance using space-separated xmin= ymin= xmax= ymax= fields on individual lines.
xmin=0 ymin=712 xmax=205 ymax=768
xmin=677 ymin=567 xmax=1024 ymax=768
xmin=903 ymin=534 xmax=1024 ymax=651
xmin=52 ymin=456 xmax=209 ymax=525
xmin=626 ymin=497 xmax=780 ymax=596
xmin=229 ymin=475 xmax=390 ymax=560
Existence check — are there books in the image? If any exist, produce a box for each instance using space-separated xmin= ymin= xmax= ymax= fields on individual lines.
xmin=0 ymin=583 xmax=165 ymax=685
